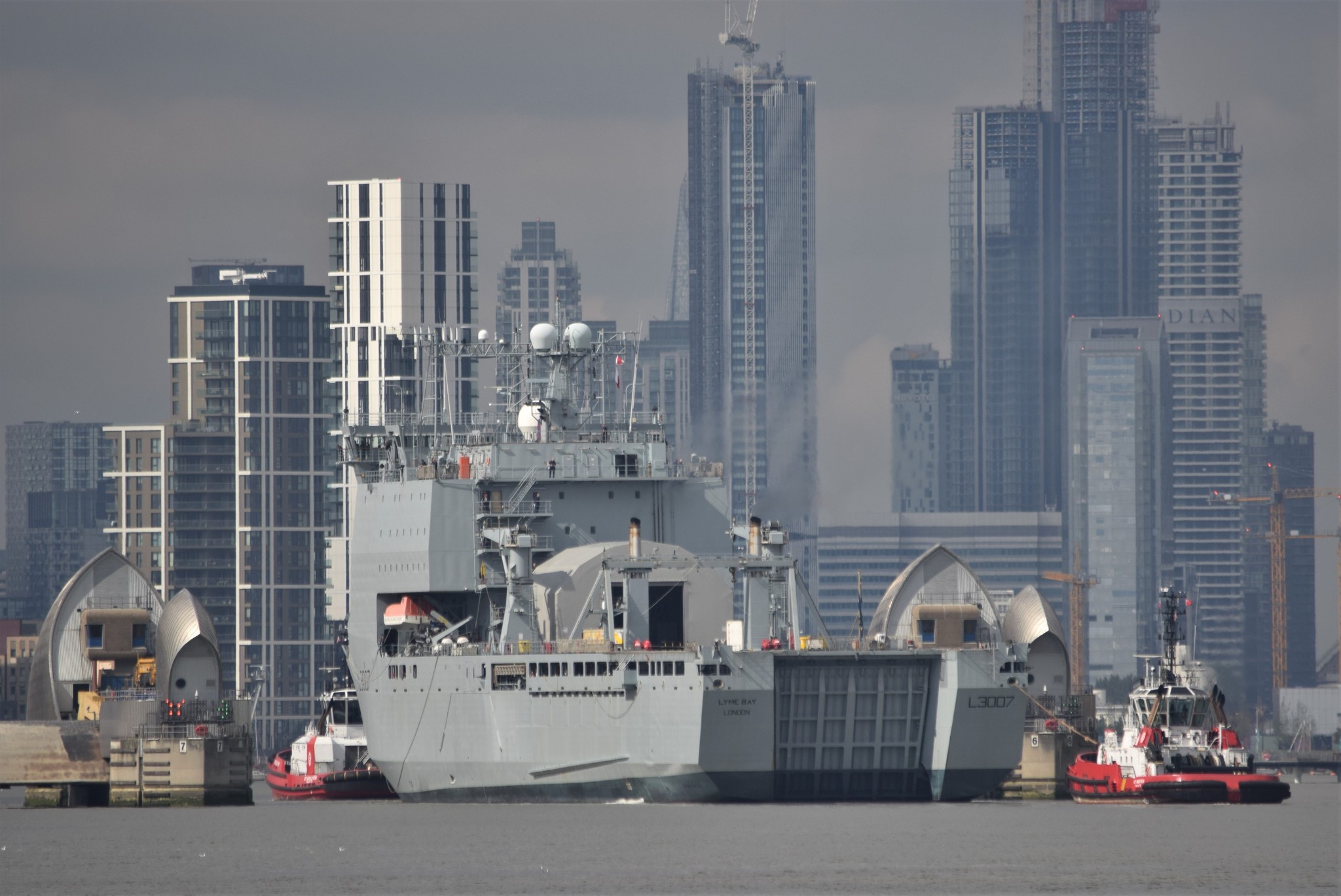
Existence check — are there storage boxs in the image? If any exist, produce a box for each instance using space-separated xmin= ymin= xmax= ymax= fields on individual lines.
xmin=581 ymin=629 xmax=623 ymax=644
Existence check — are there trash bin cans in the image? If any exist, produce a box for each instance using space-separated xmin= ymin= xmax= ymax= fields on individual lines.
xmin=800 ymin=636 xmax=809 ymax=650
xmin=519 ymin=640 xmax=530 ymax=654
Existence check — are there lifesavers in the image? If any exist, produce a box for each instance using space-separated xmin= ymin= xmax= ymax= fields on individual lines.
xmin=1046 ymin=720 xmax=1058 ymax=731
xmin=195 ymin=725 xmax=207 ymax=735
xmin=1145 ymin=768 xmax=1149 ymax=776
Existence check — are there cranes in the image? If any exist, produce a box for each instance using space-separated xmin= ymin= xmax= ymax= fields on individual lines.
xmin=1204 ymin=461 xmax=1341 ymax=750
xmin=1041 ymin=542 xmax=1102 ymax=696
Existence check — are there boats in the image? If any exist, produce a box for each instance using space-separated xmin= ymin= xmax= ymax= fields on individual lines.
xmin=1067 ymin=566 xmax=1292 ymax=806
xmin=383 ymin=594 xmax=438 ymax=631
xmin=347 ymin=320 xmax=1029 ymax=806
xmin=266 ymin=620 xmax=399 ymax=801
